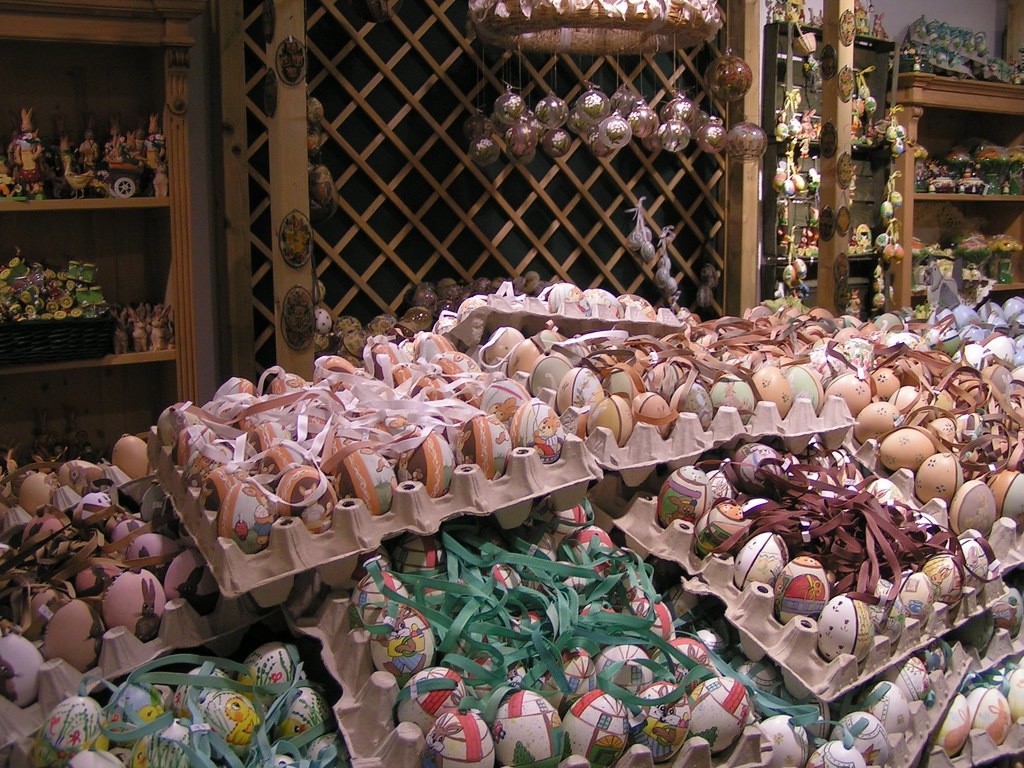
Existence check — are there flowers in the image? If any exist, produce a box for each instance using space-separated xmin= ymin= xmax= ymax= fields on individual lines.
xmin=988 ymin=234 xmax=1023 ymax=259
xmin=1006 ymin=146 xmax=1024 ymax=171
xmin=975 ymin=146 xmax=1008 ymax=174
xmin=957 ymin=231 xmax=993 ymax=263
xmin=912 ymin=236 xmax=930 ymax=273
xmin=947 ymin=152 xmax=970 ymax=174
xmin=914 ymin=143 xmax=928 ymax=176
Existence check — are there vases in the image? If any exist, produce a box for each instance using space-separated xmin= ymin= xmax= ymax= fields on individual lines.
xmin=914 ymin=176 xmax=929 ymax=193
xmin=986 ymin=174 xmax=1001 ymax=195
xmin=911 ymin=272 xmax=916 ymax=289
xmin=998 ymin=259 xmax=1013 ymax=284
xmin=1009 ymin=177 xmax=1020 ymax=195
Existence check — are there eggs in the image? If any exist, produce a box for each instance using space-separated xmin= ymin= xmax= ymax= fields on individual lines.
xmin=0 ymin=281 xmax=1024 ymax=768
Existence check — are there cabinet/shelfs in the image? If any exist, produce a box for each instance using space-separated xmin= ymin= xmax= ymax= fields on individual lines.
xmin=759 ymin=21 xmax=895 ymax=322
xmin=0 ymin=0 xmax=207 ymax=464
xmin=885 ymin=71 xmax=1024 ymax=313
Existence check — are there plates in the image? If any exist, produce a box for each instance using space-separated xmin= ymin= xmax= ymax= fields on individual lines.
xmin=818 ymin=10 xmax=857 ymax=314
